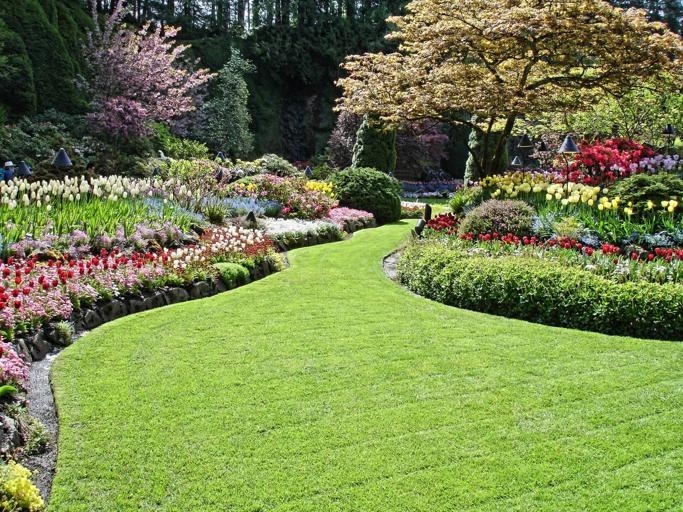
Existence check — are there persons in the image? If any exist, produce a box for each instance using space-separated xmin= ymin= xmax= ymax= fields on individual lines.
xmin=86 ymin=162 xmax=96 ymax=173
xmin=294 ymin=159 xmax=304 ymax=169
xmin=4 ymin=161 xmax=18 ymax=179
